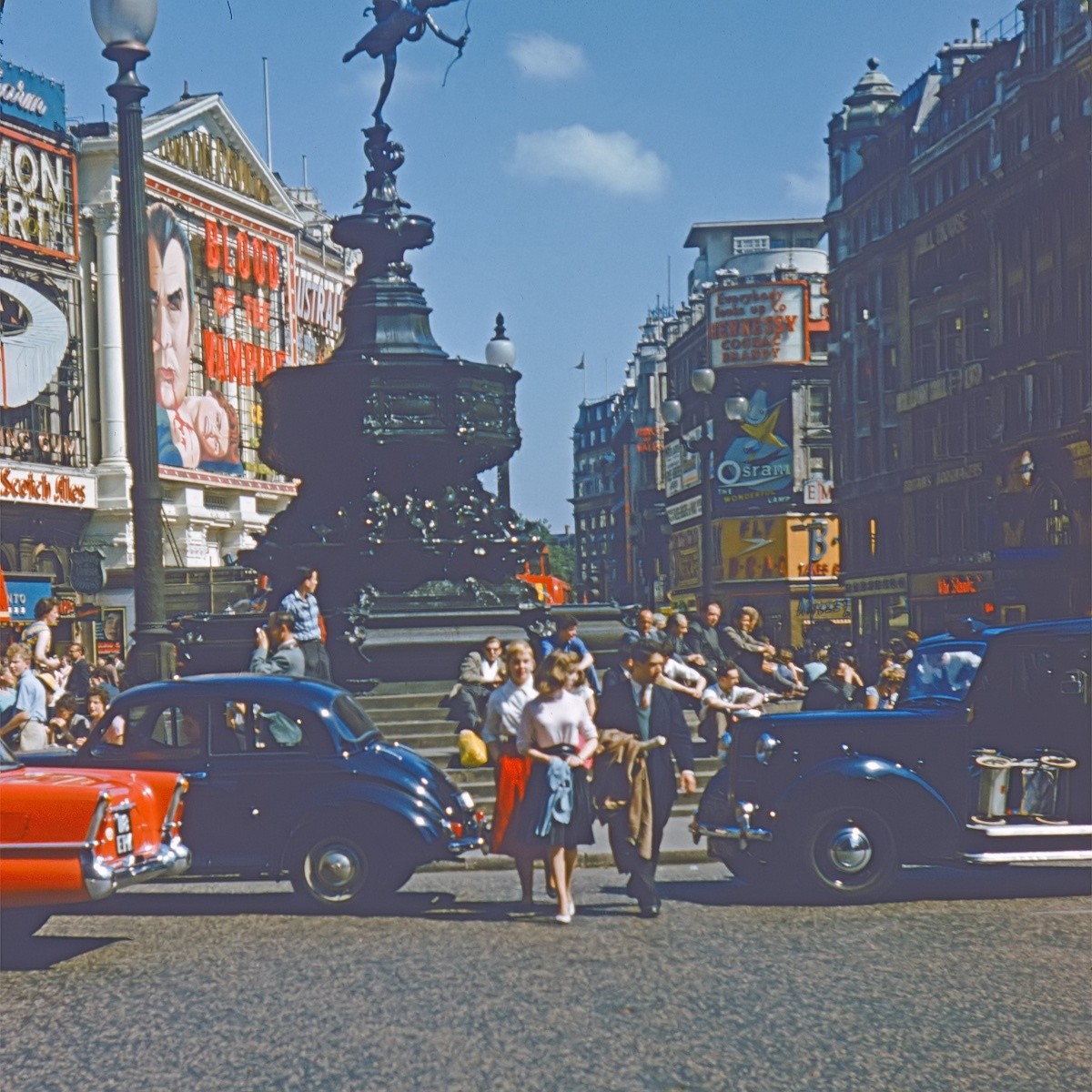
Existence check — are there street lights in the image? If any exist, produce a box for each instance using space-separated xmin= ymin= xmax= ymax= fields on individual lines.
xmin=485 ymin=312 xmax=517 ymax=509
xmin=89 ymin=0 xmax=195 ymax=748
xmin=658 ymin=350 xmax=750 ymax=613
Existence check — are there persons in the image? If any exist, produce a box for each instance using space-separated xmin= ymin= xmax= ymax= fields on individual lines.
xmin=279 ymin=569 xmax=330 ymax=679
xmin=517 ymin=649 xmax=597 ymax=920
xmin=697 ymin=663 xmax=764 ymax=760
xmin=590 ymin=638 xmax=696 ymax=916
xmin=536 ymin=616 xmax=601 ymax=695
xmin=601 ymin=602 xmax=809 ymax=708
xmin=446 ymin=637 xmax=508 ymax=732
xmin=248 ymin=612 xmax=306 ymax=748
xmin=483 ymin=638 xmax=556 ymax=909
xmin=0 ymin=597 xmax=124 ymax=751
xmin=567 ymin=651 xmax=596 ymax=769
xmin=342 ymin=0 xmax=467 ymax=123
xmin=800 ymin=628 xmax=983 ymax=709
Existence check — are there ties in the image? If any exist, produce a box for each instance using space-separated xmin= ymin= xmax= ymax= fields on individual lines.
xmin=639 ymin=685 xmax=648 ymax=711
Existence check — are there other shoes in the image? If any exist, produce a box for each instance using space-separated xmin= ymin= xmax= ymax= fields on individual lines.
xmin=567 ymin=898 xmax=576 ymax=916
xmin=544 ymin=863 xmax=559 ymax=899
xmin=555 ymin=911 xmax=571 ymax=923
xmin=640 ymin=901 xmax=660 ymax=916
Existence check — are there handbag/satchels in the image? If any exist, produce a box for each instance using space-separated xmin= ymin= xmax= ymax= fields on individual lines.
xmin=457 ymin=729 xmax=488 ymax=767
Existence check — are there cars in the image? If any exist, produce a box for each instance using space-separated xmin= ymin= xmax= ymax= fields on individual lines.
xmin=14 ymin=670 xmax=493 ymax=915
xmin=0 ymin=737 xmax=193 ymax=950
xmin=687 ymin=616 xmax=1092 ymax=906
